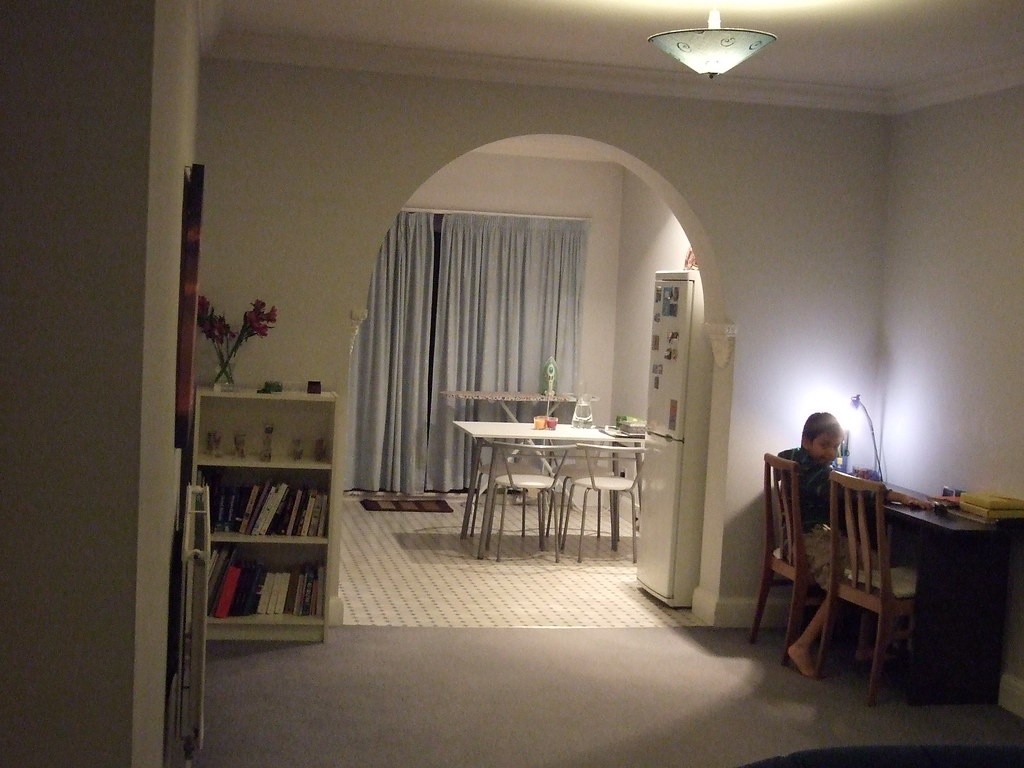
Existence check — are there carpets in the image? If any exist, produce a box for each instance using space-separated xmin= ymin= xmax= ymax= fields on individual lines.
xmin=359 ymin=499 xmax=453 ymax=513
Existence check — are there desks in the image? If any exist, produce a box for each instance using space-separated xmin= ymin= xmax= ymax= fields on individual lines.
xmin=857 ymin=484 xmax=1010 ymax=707
xmin=453 ymin=419 xmax=649 ymax=561
xmin=441 ymin=390 xmax=599 ymax=516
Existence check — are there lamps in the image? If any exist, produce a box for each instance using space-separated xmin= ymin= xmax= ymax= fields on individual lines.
xmin=851 ymin=395 xmax=884 ymax=479
xmin=649 ymin=9 xmax=778 ymax=81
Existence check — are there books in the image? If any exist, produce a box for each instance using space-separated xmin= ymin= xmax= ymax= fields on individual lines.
xmin=208 ymin=550 xmax=323 ymax=618
xmin=208 ymin=479 xmax=326 ymax=538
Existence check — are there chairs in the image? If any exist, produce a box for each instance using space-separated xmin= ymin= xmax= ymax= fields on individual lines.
xmin=815 ymin=470 xmax=917 ymax=701
xmin=749 ymin=454 xmax=814 ymax=665
xmin=489 ymin=439 xmax=575 ymax=562
xmin=577 ymin=443 xmax=646 ymax=565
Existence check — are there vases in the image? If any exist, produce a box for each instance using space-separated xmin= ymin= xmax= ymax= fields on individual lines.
xmin=211 ymin=362 xmax=243 ymax=391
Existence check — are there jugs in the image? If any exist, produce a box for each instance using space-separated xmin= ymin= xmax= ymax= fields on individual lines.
xmin=571 ymin=393 xmax=593 ymax=429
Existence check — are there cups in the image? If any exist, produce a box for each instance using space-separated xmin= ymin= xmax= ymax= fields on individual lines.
xmin=534 ymin=416 xmax=546 ymax=430
xmin=546 ymin=417 xmax=559 ymax=431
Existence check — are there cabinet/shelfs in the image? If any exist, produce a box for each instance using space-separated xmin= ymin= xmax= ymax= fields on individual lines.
xmin=191 ymin=385 xmax=342 ymax=642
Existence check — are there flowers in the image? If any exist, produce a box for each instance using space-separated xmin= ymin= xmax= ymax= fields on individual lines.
xmin=195 ymin=293 xmax=277 ymax=360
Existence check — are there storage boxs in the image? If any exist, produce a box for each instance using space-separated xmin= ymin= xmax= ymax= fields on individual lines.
xmin=959 ymin=503 xmax=1024 ymax=519
xmin=959 ymin=492 xmax=1024 ymax=510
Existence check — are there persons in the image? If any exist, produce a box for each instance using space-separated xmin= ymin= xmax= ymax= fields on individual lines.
xmin=774 ymin=410 xmax=932 ymax=679
